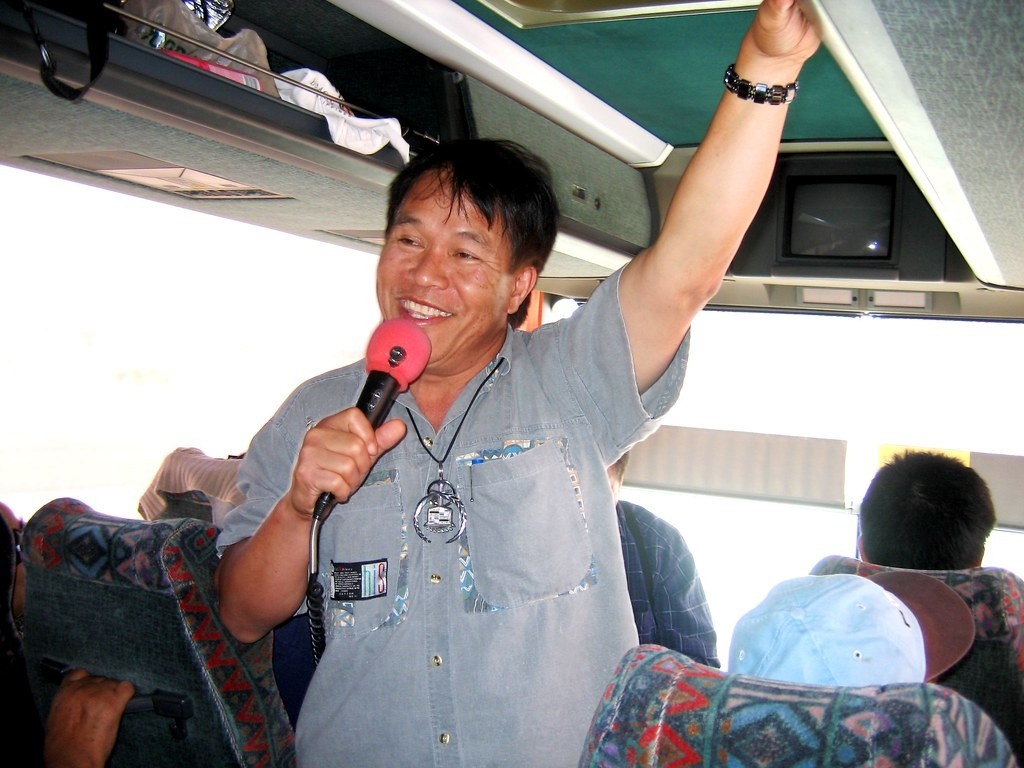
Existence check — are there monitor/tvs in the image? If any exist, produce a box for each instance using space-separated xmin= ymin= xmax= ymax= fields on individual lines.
xmin=783 ymin=174 xmax=896 ymax=260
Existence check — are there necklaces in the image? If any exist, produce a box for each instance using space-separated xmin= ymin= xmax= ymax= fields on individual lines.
xmin=405 ymin=358 xmax=503 ymax=544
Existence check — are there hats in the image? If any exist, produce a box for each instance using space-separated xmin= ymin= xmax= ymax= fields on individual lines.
xmin=728 ymin=571 xmax=976 ymax=686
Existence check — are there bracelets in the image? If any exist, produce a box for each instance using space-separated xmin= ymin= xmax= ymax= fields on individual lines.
xmin=725 ymin=63 xmax=799 ymax=105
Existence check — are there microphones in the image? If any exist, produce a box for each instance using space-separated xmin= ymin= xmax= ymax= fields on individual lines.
xmin=313 ymin=317 xmax=433 ymax=522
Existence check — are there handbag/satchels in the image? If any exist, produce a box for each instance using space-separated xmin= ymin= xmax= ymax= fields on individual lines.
xmin=124 ymin=0 xmax=281 ymax=105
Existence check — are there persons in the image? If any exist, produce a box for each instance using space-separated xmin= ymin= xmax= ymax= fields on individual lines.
xmin=215 ymin=0 xmax=822 ymax=768
xmin=0 ymin=503 xmax=133 ymax=768
xmin=607 ymin=451 xmax=721 ymax=669
xmin=728 ymin=571 xmax=974 ymax=687
xmin=858 ymin=451 xmax=995 ymax=570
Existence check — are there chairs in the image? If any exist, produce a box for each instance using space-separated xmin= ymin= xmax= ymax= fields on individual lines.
xmin=577 ymin=644 xmax=1019 ymax=768
xmin=0 ymin=499 xmax=299 ymax=768
xmin=809 ymin=555 xmax=1024 ymax=768
